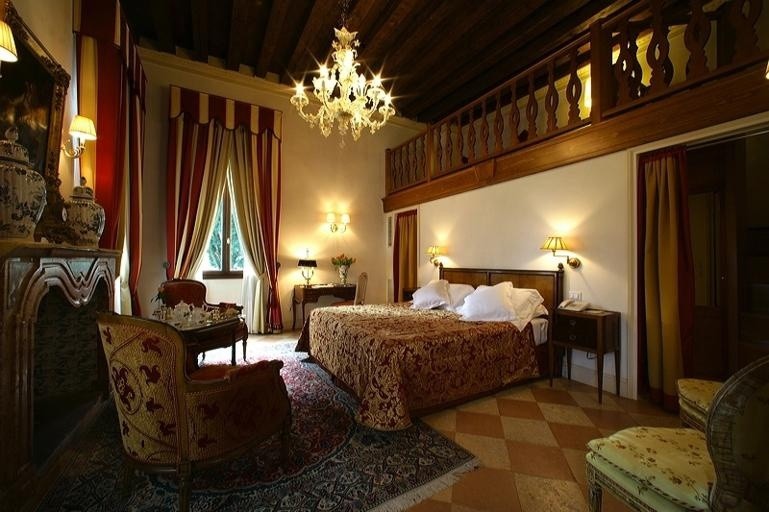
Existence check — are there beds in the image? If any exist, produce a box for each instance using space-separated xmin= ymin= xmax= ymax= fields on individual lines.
xmin=292 ymin=264 xmax=564 ymax=432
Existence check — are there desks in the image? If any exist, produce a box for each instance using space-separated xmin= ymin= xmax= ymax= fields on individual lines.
xmin=143 ymin=309 xmax=241 ymax=369
xmin=293 ymin=283 xmax=356 ymax=331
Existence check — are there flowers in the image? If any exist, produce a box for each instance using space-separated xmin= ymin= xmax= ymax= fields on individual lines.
xmin=331 ymin=253 xmax=356 ymax=269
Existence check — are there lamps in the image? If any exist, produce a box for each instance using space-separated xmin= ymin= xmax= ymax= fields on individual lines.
xmin=296 ymin=258 xmax=317 ymax=288
xmin=425 ymin=244 xmax=444 ymax=269
xmin=61 ymin=116 xmax=97 ymax=159
xmin=0 ymin=3 xmax=23 ymax=66
xmin=285 ymin=5 xmax=398 ymax=148
xmin=539 ymin=234 xmax=581 ymax=271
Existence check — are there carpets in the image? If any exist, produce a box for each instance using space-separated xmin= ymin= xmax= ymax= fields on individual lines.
xmin=16 ymin=351 xmax=480 ymax=510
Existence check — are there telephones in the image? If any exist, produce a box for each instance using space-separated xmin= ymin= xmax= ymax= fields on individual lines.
xmin=557 ymin=299 xmax=590 ymax=311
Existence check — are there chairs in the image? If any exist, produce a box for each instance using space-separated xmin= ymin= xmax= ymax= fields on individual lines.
xmin=584 ymin=353 xmax=769 ymax=511
xmin=157 ymin=277 xmax=249 ymax=366
xmin=88 ymin=307 xmax=292 ymax=510
xmin=674 ymin=374 xmax=725 ymax=431
xmin=331 ymin=271 xmax=369 ymax=307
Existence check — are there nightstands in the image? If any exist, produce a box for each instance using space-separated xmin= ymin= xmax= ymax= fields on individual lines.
xmin=547 ymin=307 xmax=620 ymax=406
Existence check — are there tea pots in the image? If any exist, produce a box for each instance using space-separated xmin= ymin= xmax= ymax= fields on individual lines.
xmin=175 ymin=300 xmax=191 ymax=319
xmin=192 ymin=303 xmax=208 ymax=322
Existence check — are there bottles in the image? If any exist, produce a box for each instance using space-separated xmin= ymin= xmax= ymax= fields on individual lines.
xmin=164 ymin=305 xmax=172 ymax=319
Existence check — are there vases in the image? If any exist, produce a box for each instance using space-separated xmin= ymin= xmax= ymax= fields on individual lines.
xmin=336 ymin=263 xmax=350 ymax=286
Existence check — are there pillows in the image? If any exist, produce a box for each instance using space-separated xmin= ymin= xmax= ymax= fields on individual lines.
xmin=402 ymin=276 xmax=545 ymax=330
